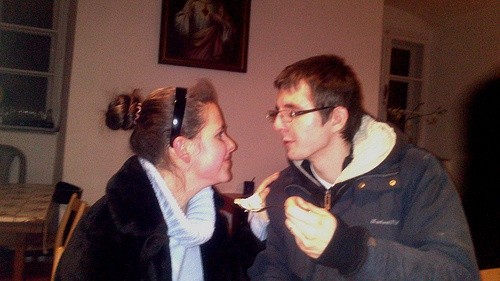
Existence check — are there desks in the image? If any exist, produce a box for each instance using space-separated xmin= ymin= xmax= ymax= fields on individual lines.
xmin=0 ymin=183 xmax=56 ymax=281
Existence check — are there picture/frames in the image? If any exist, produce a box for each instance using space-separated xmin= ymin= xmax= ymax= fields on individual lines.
xmin=158 ymin=0 xmax=251 ymax=73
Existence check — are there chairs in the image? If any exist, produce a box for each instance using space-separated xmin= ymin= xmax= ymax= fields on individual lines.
xmin=0 ymin=144 xmax=26 ymax=184
xmin=22 ymin=181 xmax=89 ymax=281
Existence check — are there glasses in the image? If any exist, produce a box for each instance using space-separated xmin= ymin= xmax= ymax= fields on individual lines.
xmin=265 ymin=106 xmax=334 ymax=123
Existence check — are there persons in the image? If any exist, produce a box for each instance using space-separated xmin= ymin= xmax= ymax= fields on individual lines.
xmin=54 ymin=79 xmax=278 ymax=281
xmin=247 ymin=56 xmax=478 ymax=281
